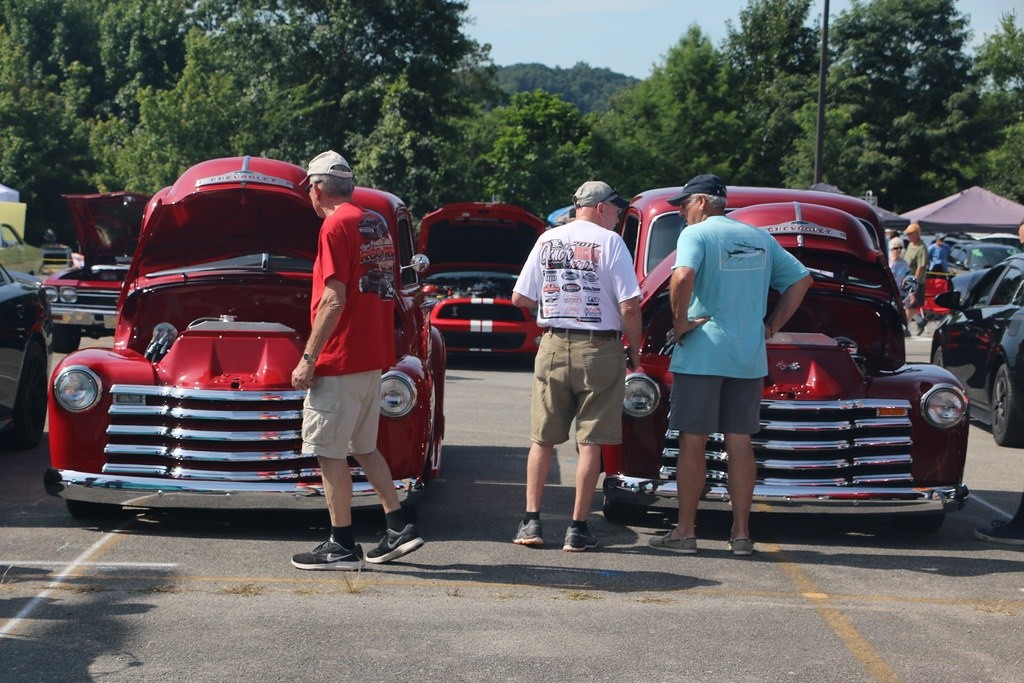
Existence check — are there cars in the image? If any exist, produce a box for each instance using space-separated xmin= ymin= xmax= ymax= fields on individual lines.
xmin=594 ymin=182 xmax=971 ymax=537
xmin=42 ymin=154 xmax=448 ymax=526
xmin=871 ymin=205 xmax=1024 ymax=320
xmin=546 ymin=197 xmax=632 ymax=234
xmin=411 ymin=202 xmax=563 ymax=365
xmin=927 ymin=253 xmax=1024 ymax=446
xmin=0 ymin=222 xmax=45 ymax=274
xmin=0 ymin=259 xmax=55 ymax=451
xmin=40 ymin=190 xmax=151 ymax=355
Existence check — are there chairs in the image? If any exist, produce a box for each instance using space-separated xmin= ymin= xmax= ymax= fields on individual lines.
xmin=916 ymin=279 xmax=950 ymax=322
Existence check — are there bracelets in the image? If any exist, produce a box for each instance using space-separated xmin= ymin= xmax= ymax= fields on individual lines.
xmin=770 ymin=327 xmax=774 ymax=337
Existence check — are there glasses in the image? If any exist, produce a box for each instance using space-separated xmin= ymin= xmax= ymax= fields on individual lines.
xmin=307 ymin=180 xmax=323 ymax=191
xmin=894 ymin=247 xmax=902 ymax=250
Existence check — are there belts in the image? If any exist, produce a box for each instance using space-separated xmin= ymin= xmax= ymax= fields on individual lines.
xmin=545 ymin=327 xmax=622 ymax=338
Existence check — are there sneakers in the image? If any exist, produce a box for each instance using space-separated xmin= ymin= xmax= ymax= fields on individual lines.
xmin=730 ymin=539 xmax=753 ymax=555
xmin=563 ymin=527 xmax=598 ymax=550
xmin=512 ymin=520 xmax=544 ymax=544
xmin=649 ymin=532 xmax=697 ymax=553
xmin=291 ymin=538 xmax=365 ymax=570
xmin=365 ymin=523 xmax=423 ymax=563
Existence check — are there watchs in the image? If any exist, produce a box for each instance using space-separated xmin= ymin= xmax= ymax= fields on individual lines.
xmin=303 ymin=352 xmax=317 ymax=363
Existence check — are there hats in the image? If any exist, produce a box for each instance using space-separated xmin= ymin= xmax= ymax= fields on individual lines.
xmin=667 ymin=174 xmax=726 ymax=206
xmin=903 ymin=223 xmax=921 ymax=235
xmin=299 ymin=150 xmax=353 ymax=186
xmin=937 ymin=233 xmax=947 ymax=239
xmin=573 ymin=181 xmax=630 ymax=209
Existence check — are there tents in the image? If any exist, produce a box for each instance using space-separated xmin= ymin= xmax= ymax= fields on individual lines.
xmin=810 ymin=183 xmax=912 ymax=231
xmin=901 ymin=185 xmax=1024 ymax=233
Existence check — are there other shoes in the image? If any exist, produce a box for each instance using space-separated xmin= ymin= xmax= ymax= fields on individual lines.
xmin=904 ymin=330 xmax=911 ymax=337
xmin=916 ymin=319 xmax=928 ymax=336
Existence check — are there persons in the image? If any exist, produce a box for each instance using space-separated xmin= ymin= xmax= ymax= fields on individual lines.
xmin=903 ymin=224 xmax=929 ymax=337
xmin=927 ymin=231 xmax=951 ymax=272
xmin=291 ymin=150 xmax=423 ymax=571
xmin=889 ymin=238 xmax=910 ymax=298
xmin=510 ymin=181 xmax=645 ymax=553
xmin=884 ymin=229 xmax=897 ymax=257
xmin=649 ymin=174 xmax=815 ymax=554
